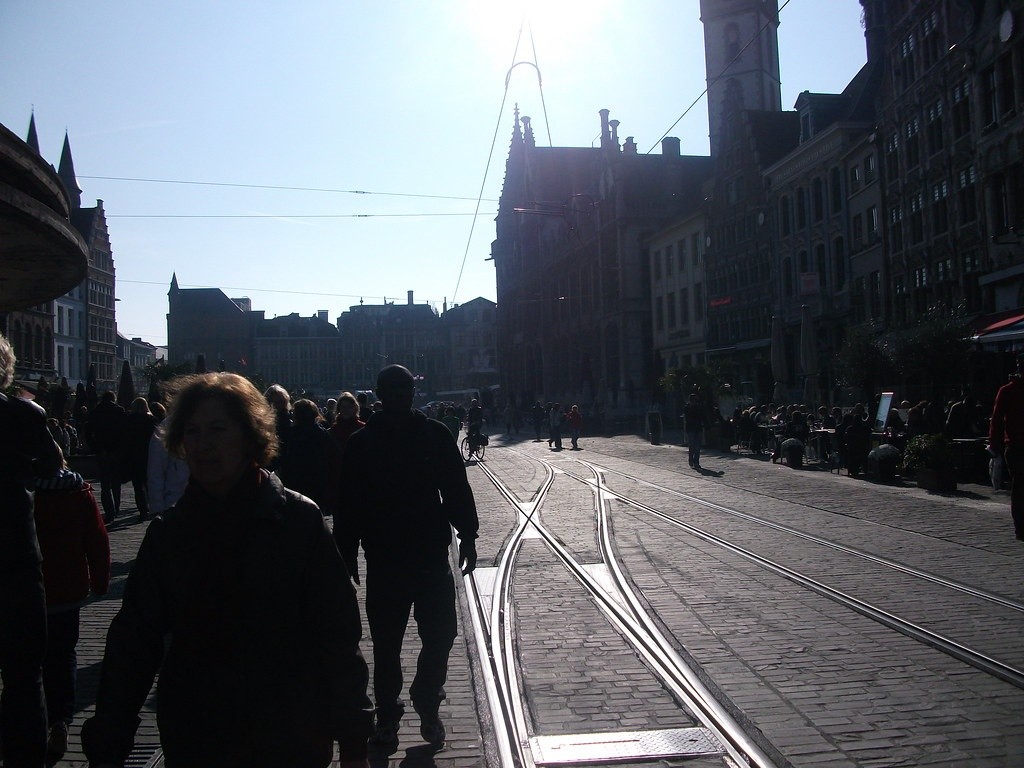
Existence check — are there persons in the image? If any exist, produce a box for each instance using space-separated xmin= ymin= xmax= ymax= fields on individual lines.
xmin=441 ymin=405 xmax=460 ymax=444
xmin=327 ymin=389 xmax=368 ymax=441
xmin=76 ymin=379 xmax=85 ymax=394
xmin=266 ymin=381 xmax=290 ymax=457
xmin=283 ymin=396 xmax=332 ymax=519
xmin=292 ymin=387 xmax=450 ymax=429
xmin=87 ymin=387 xmax=127 ymax=521
xmin=46 ymin=413 xmax=80 ymax=457
xmin=82 ymin=372 xmax=381 ymax=768
xmin=37 ymin=375 xmax=48 ymax=391
xmin=35 ymin=416 xmax=111 ymax=754
xmin=0 ymin=328 xmax=62 ymax=768
xmin=986 ymin=353 xmax=1023 ymax=540
xmin=333 ymin=365 xmax=480 ymax=749
xmin=732 ymin=391 xmax=984 ymax=464
xmin=683 ymin=394 xmax=702 ymax=468
xmin=483 ymin=400 xmax=580 ymax=450
xmin=467 ymin=397 xmax=482 ymax=456
xmin=124 ymin=394 xmax=155 ymax=516
xmin=60 ymin=376 xmax=69 ymax=389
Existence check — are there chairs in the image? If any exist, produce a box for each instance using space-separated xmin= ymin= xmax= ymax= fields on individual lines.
xmin=825 ymin=449 xmax=846 ymax=475
xmin=735 ymin=425 xmax=766 ymax=458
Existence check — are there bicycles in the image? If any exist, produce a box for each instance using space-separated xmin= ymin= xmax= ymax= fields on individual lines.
xmin=461 ymin=422 xmax=485 ymax=461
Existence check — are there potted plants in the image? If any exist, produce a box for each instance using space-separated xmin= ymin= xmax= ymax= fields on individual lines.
xmin=903 ymin=431 xmax=961 ymax=493
xmin=780 ymin=436 xmax=805 ymax=469
xmin=865 ymin=443 xmax=903 ymax=486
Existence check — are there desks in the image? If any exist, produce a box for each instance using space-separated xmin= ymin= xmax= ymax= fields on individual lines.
xmin=814 ymin=428 xmax=835 ymax=463
xmin=870 ymin=431 xmax=900 ymax=447
xmin=952 ymin=437 xmax=990 ymax=483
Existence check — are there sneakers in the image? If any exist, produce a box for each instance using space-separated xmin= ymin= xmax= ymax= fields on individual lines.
xmin=45 ymin=721 xmax=70 ymax=762
xmin=411 ymin=696 xmax=446 ymax=744
xmin=369 ymin=714 xmax=401 ymax=748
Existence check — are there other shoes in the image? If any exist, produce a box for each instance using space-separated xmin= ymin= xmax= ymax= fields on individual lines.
xmin=1015 ymin=533 xmax=1024 ymax=542
xmin=693 ymin=460 xmax=701 ymax=468
xmin=689 ymin=457 xmax=694 ymax=467
xmin=114 ymin=509 xmax=119 ymax=517
xmin=105 ymin=513 xmax=114 ymax=522
xmin=139 ymin=511 xmax=156 ymax=520
